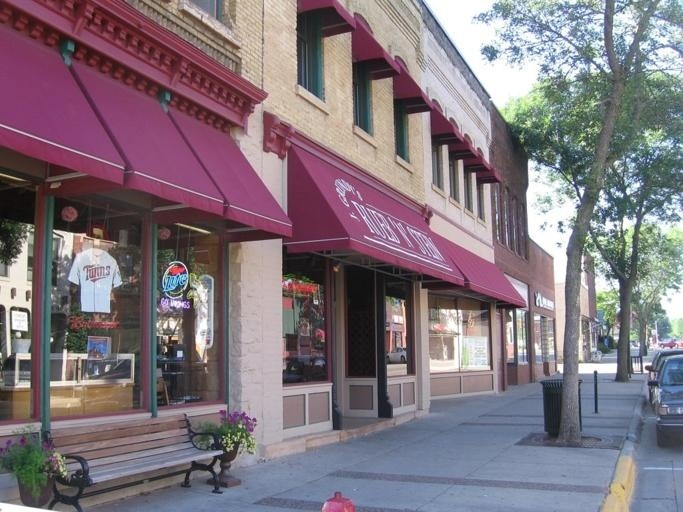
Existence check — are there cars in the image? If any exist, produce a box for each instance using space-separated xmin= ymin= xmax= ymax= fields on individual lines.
xmin=645 ymin=348 xmax=683 ymax=448
xmin=658 ymin=338 xmax=678 ymax=348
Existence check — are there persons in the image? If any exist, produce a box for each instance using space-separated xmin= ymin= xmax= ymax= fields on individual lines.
xmin=604 ymin=336 xmax=608 ymax=348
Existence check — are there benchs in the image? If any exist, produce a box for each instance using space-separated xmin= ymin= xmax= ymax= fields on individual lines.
xmin=42 ymin=413 xmax=224 ymax=512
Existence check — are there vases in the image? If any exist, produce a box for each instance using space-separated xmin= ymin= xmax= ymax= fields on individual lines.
xmin=16 ymin=474 xmax=54 ymax=507
xmin=207 ymin=441 xmax=241 ymax=488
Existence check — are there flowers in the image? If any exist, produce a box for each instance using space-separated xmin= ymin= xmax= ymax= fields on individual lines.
xmin=0 ymin=404 xmax=67 ymax=500
xmin=189 ymin=410 xmax=257 ymax=455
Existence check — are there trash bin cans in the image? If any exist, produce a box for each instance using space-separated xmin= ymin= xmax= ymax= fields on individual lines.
xmin=540 ymin=379 xmax=583 ymax=436
